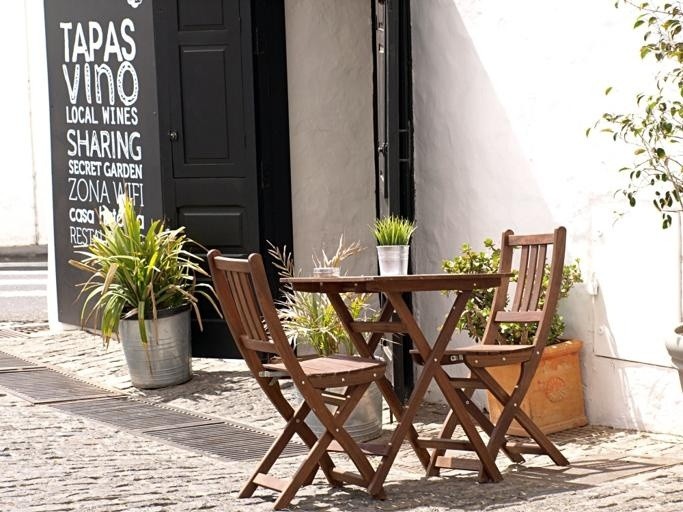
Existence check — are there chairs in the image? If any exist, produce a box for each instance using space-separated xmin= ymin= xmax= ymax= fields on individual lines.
xmin=407 ymin=225 xmax=570 ymax=485
xmin=207 ymin=249 xmax=388 ymax=511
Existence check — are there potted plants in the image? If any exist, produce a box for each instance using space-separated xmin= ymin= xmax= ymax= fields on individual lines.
xmin=442 ymin=238 xmax=588 ymax=438
xmin=586 ymin=0 xmax=683 ymax=394
xmin=67 ymin=178 xmax=225 ymax=391
xmin=367 ymin=212 xmax=419 ymax=277
xmin=261 ymin=232 xmax=404 ymax=445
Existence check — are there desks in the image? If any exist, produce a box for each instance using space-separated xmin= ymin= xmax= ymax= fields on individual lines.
xmin=275 ymin=272 xmax=516 ymax=503
